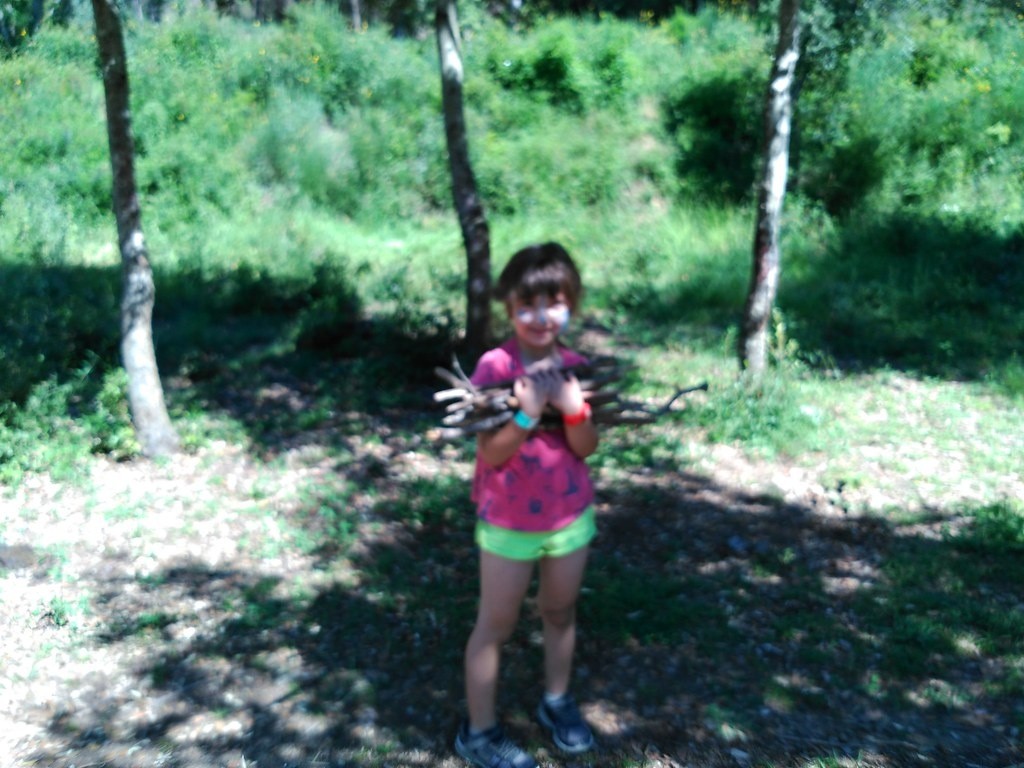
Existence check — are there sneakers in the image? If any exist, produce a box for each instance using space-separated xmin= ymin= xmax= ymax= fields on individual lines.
xmin=454 ymin=721 xmax=539 ymax=768
xmin=535 ymin=691 xmax=594 ymax=754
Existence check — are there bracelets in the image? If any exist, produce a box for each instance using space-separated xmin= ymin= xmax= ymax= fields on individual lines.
xmin=513 ymin=410 xmax=540 ymax=429
xmin=564 ymin=402 xmax=591 ymax=426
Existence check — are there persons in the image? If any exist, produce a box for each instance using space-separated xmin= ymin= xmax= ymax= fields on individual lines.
xmin=455 ymin=240 xmax=606 ymax=768
